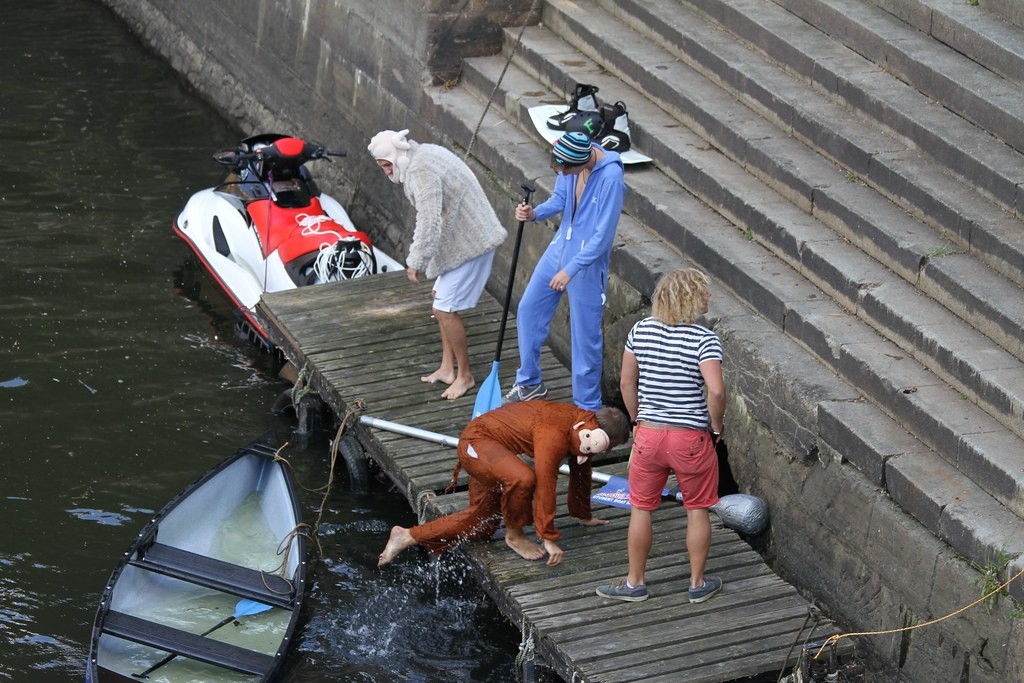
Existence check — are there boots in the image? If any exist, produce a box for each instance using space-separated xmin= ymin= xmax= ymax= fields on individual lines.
xmin=547 ymin=84 xmax=599 ymax=130
xmin=592 ymin=100 xmax=631 ymax=152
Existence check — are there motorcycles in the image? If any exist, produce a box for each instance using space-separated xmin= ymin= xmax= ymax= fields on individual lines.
xmin=172 ymin=132 xmax=405 ymax=362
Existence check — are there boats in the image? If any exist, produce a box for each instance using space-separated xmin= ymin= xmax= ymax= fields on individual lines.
xmin=83 ymin=419 xmax=313 ymax=683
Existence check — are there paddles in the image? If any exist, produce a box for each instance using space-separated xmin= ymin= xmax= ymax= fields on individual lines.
xmin=131 ymin=597 xmax=273 ymax=679
xmin=471 ymin=186 xmax=535 ymax=423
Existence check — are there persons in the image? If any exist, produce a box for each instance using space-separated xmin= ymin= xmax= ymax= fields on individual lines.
xmin=366 ymin=129 xmax=508 ymax=400
xmin=596 ymin=269 xmax=727 ymax=605
xmin=503 ymin=132 xmax=627 ymax=411
xmin=380 ymin=400 xmax=630 ymax=566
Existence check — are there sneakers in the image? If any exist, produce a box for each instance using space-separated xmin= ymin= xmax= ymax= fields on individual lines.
xmin=688 ymin=574 xmax=722 ymax=602
xmin=596 ymin=580 xmax=649 ymax=602
xmin=503 ymin=382 xmax=549 ymax=406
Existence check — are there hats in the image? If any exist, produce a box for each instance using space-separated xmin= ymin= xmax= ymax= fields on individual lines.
xmin=553 ymin=131 xmax=593 ymax=164
xmin=368 ymin=127 xmax=410 ymax=183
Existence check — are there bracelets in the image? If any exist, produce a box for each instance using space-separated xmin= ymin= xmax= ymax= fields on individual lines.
xmin=709 ymin=422 xmax=724 ymax=435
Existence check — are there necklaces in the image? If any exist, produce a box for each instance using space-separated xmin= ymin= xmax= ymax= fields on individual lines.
xmin=583 ymin=148 xmax=597 ymax=184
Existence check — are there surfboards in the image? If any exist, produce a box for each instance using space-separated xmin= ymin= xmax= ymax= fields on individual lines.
xmin=529 ymin=103 xmax=654 ymax=165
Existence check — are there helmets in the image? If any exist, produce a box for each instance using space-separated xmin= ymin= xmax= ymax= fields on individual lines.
xmin=566 ymin=111 xmax=604 ymax=140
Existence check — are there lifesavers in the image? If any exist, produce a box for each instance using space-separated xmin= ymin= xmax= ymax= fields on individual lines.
xmin=325 ymin=434 xmax=376 ymax=492
xmin=270 ymin=386 xmax=324 ymax=434
xmin=512 ymin=653 xmax=540 ymax=682
